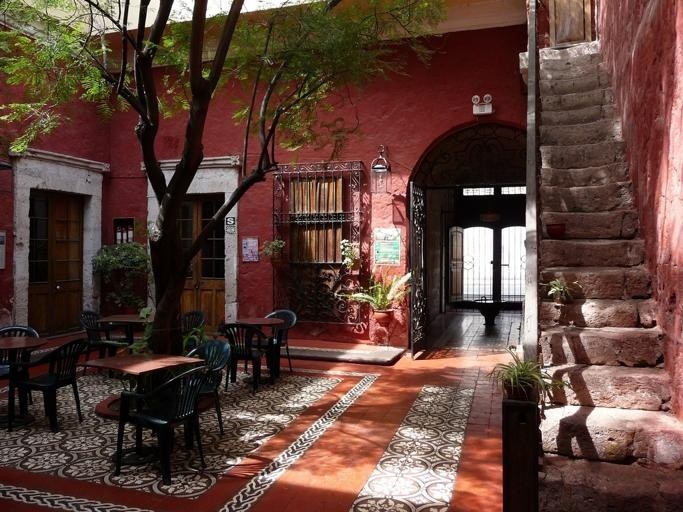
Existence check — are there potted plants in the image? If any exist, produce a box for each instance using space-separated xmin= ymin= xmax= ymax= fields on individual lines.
xmin=335 ymin=262 xmax=412 ymax=323
xmin=487 ymin=347 xmax=569 ymax=400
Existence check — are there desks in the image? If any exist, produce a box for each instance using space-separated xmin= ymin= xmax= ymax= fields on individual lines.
xmin=2 ymin=335 xmax=49 ymax=424
xmin=79 ymin=352 xmax=206 ymax=464
xmin=224 ymin=317 xmax=285 ymax=384
xmin=98 ymin=311 xmax=157 ymax=352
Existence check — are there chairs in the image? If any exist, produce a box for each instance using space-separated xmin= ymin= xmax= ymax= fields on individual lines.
xmin=78 ymin=311 xmax=130 ymax=375
xmin=260 ymin=310 xmax=296 ymax=374
xmin=22 ymin=336 xmax=88 ymax=427
xmin=218 ymin=323 xmax=274 ymax=391
xmin=113 ymin=363 xmax=208 ymax=485
xmin=1 ymin=325 xmax=40 ymax=414
xmin=165 ymin=341 xmax=232 ymax=440
xmin=180 ymin=310 xmax=206 ymax=341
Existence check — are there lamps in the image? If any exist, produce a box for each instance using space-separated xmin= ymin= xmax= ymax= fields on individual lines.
xmin=370 ymin=143 xmax=393 ymax=196
xmin=472 ymin=93 xmax=494 ymax=115
